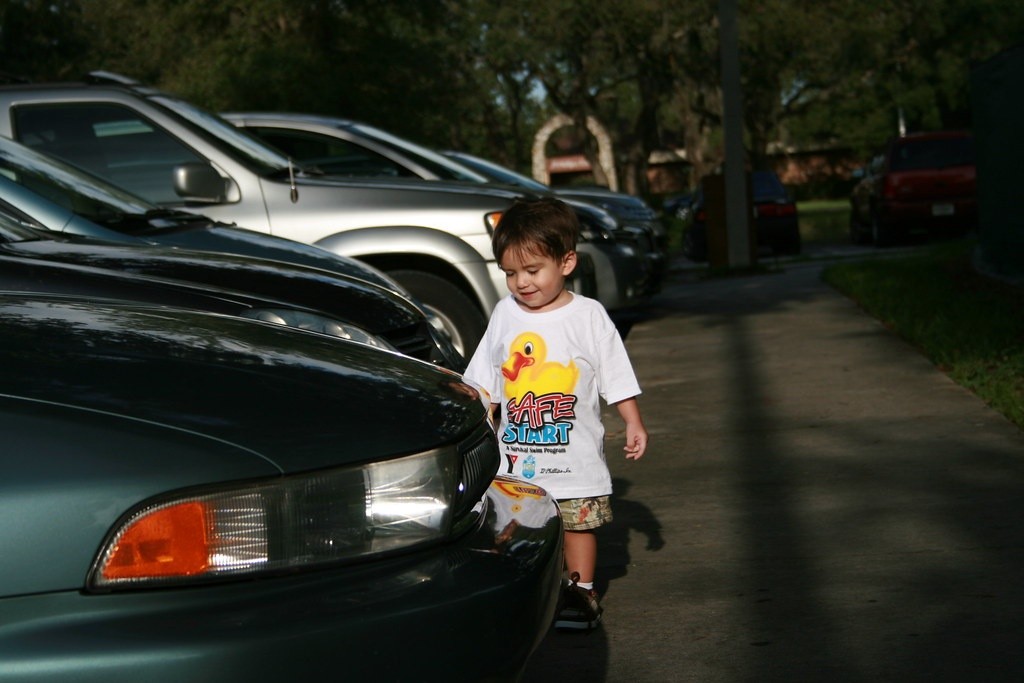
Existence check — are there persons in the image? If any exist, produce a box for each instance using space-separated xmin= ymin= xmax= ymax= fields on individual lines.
xmin=462 ymin=197 xmax=648 ymax=630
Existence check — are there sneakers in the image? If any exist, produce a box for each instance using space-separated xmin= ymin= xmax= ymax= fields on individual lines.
xmin=552 ymin=571 xmax=601 ymax=631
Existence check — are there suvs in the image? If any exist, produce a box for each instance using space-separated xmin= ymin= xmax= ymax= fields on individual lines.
xmin=0 ymin=70 xmax=588 ymax=393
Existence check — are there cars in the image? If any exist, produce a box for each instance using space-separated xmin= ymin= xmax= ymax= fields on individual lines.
xmin=0 ymin=132 xmax=468 ymax=380
xmin=679 ymin=174 xmax=803 ymax=264
xmin=0 ymin=245 xmax=561 ymax=683
xmin=17 ymin=109 xmax=670 ymax=339
xmin=846 ymin=129 xmax=976 ymax=248
